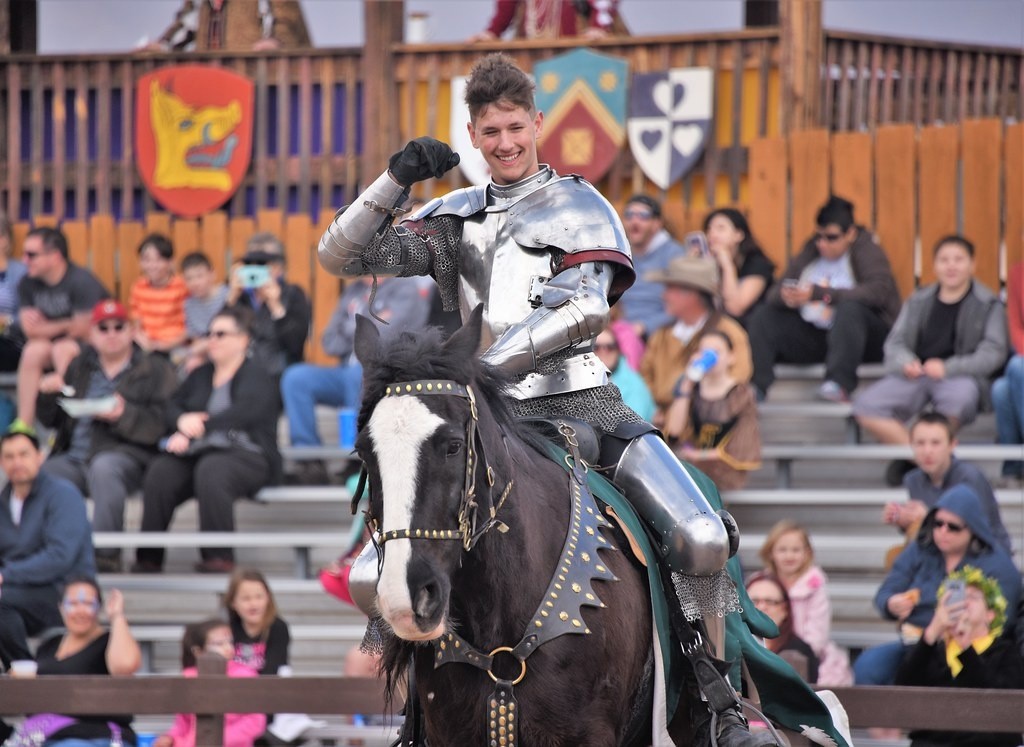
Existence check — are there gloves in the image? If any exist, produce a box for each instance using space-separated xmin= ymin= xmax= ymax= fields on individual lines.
xmin=387 ymin=134 xmax=461 ymax=184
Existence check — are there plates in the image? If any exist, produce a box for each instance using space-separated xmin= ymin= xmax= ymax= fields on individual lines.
xmin=55 ymin=395 xmax=117 ymax=417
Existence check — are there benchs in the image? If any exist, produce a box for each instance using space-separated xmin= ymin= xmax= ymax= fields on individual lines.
xmin=85 ymin=365 xmax=1024 ymax=747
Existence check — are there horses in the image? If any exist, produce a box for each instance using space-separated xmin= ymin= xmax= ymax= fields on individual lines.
xmin=339 ymin=304 xmax=714 ymax=747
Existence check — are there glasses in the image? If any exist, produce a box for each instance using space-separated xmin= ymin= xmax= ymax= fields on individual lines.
xmin=749 ymin=597 xmax=787 ymax=609
xmin=595 ymin=341 xmax=618 ymax=354
xmin=98 ymin=324 xmax=126 ymax=333
xmin=25 ymin=251 xmax=56 ymax=260
xmin=624 ymin=210 xmax=657 ymax=223
xmin=932 ymin=517 xmax=969 ymax=532
xmin=242 ymin=257 xmax=271 ymax=269
xmin=812 ymin=229 xmax=852 ymax=242
xmin=204 ymin=330 xmax=230 ymax=339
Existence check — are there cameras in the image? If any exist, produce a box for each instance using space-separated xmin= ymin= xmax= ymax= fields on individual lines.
xmin=234 ymin=265 xmax=269 ymax=287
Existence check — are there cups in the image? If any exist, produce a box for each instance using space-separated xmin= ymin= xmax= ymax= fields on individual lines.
xmin=685 ymin=349 xmax=718 ymax=382
xmin=337 ymin=410 xmax=358 ymax=451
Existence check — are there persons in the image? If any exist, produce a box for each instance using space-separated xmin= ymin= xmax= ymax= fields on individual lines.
xmin=743 ymin=195 xmax=901 ymax=402
xmin=987 ymin=261 xmax=1024 ymax=481
xmin=465 ymin=0 xmax=628 ymax=46
xmin=851 ymin=483 xmax=1024 ymax=742
xmin=1 ymin=567 xmax=408 ymax=747
xmin=0 ymin=431 xmax=98 ymax=673
xmin=127 ymin=314 xmax=282 ymax=573
xmin=311 ymin=52 xmax=752 ymax=747
xmin=891 ymin=564 xmax=1024 ymax=747
xmin=139 ymin=0 xmax=312 ymax=51
xmin=615 ymin=196 xmax=692 ymax=337
xmin=592 ymin=300 xmax=661 ymax=425
xmin=851 ymin=235 xmax=1008 ymax=487
xmin=700 ymin=207 xmax=777 ymax=329
xmin=747 ymin=517 xmax=855 ymax=686
xmin=882 ymin=411 xmax=1011 ymax=556
xmin=0 ymin=212 xmax=426 ymax=484
xmin=34 ymin=299 xmax=178 ymax=572
xmin=739 ymin=570 xmax=819 ymax=684
xmin=636 ymin=252 xmax=761 ymax=495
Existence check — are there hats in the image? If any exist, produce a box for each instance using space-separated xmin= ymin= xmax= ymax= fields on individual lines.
xmin=233 ymin=234 xmax=286 ymax=264
xmin=92 ymin=301 xmax=134 ymax=324
xmin=642 ymin=254 xmax=720 ymax=298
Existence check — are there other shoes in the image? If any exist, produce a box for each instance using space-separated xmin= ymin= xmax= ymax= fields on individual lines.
xmin=696 ymin=707 xmax=772 ymax=747
xmin=819 ymin=379 xmax=848 ymax=400
xmin=197 ymin=556 xmax=232 ymax=573
xmin=88 ymin=553 xmax=126 ymax=573
xmin=288 ymin=464 xmax=328 ymax=486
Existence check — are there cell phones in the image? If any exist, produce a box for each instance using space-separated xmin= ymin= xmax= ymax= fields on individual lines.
xmin=684 ymin=231 xmax=707 ymax=257
xmin=783 ymin=283 xmax=799 ymax=292
